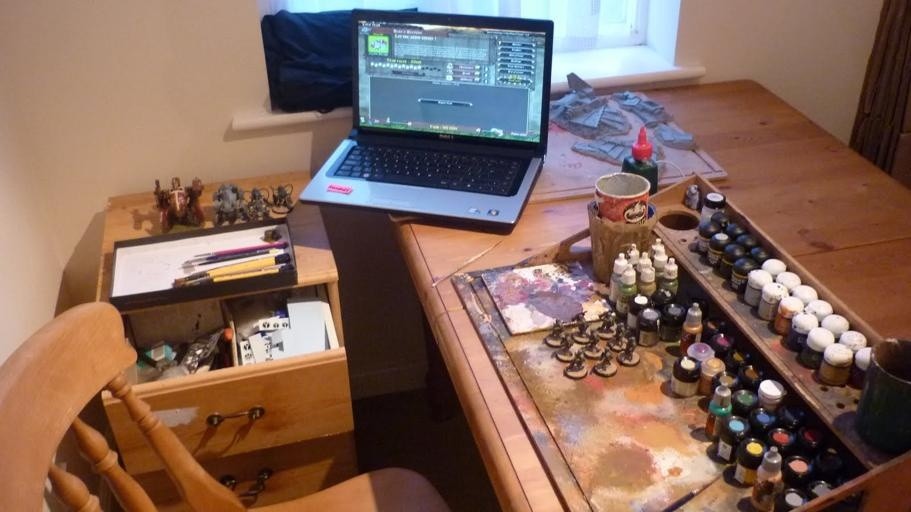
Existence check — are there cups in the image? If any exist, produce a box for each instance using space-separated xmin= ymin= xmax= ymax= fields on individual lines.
xmin=594 ymin=172 xmax=651 ymax=223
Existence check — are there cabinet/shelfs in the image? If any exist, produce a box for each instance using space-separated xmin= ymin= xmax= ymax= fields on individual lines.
xmin=93 ymin=171 xmax=358 ymax=511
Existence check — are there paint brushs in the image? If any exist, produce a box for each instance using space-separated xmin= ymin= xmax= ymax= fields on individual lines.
xmin=432 ymin=239 xmax=502 ymax=288
xmin=662 ymin=464 xmax=733 ymax=512
xmin=173 ymin=252 xmax=295 ymax=288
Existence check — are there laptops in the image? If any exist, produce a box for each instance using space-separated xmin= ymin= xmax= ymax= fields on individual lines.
xmin=300 ymin=8 xmax=552 ymax=230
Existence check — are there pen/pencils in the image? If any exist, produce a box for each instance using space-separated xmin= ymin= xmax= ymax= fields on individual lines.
xmin=182 ymin=241 xmax=289 ymax=269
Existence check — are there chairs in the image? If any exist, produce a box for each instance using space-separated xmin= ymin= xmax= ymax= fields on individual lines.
xmin=0 ymin=301 xmax=453 ymax=512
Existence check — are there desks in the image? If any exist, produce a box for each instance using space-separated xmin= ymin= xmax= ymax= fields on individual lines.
xmin=393 ymin=81 xmax=911 ymax=512
